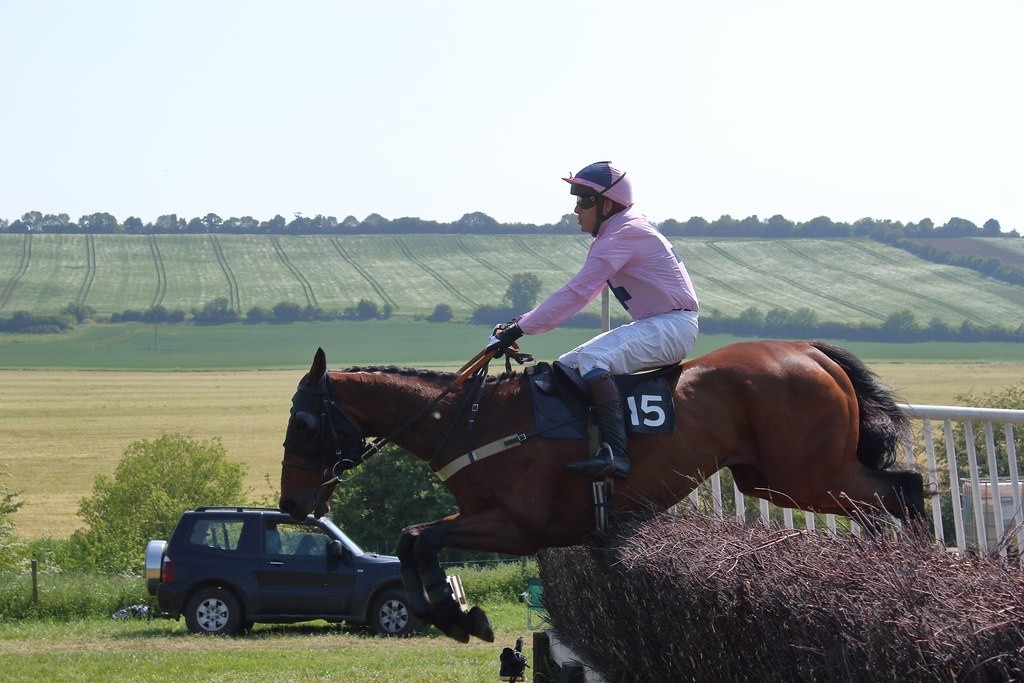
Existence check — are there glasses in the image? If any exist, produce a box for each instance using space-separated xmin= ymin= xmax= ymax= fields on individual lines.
xmin=577 ymin=172 xmax=626 ymax=210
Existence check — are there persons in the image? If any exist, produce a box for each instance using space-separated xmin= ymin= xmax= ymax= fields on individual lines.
xmin=294 ymin=534 xmax=316 ymax=555
xmin=485 ymin=162 xmax=700 ymax=478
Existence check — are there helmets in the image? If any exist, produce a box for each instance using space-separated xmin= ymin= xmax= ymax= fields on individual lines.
xmin=561 ymin=160 xmax=633 ymax=208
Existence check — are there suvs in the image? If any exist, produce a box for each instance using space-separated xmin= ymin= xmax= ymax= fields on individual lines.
xmin=146 ymin=505 xmax=432 ymax=639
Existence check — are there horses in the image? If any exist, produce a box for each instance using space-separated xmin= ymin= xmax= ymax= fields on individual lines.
xmin=277 ymin=339 xmax=926 ymax=645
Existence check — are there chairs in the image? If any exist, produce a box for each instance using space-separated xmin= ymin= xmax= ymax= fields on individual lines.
xmin=267 ymin=530 xmax=282 ymax=554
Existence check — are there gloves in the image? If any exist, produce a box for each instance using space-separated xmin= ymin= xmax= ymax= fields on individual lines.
xmin=484 ymin=317 xmax=524 ymax=358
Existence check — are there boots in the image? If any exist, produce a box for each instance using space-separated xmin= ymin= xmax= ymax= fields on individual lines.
xmin=565 ymin=372 xmax=632 ymax=483
